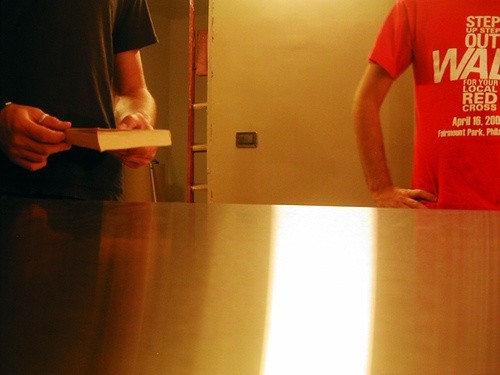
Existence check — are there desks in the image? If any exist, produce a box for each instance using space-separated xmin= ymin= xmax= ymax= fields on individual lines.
xmin=1 ymin=200 xmax=499 ymax=375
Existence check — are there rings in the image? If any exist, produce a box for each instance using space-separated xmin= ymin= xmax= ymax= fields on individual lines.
xmin=38 ymin=114 xmax=49 ymax=125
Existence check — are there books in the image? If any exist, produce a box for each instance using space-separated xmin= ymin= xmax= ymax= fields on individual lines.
xmin=63 ymin=127 xmax=173 ymax=153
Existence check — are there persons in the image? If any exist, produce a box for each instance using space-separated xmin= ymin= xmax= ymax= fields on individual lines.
xmin=349 ymin=0 xmax=500 ymax=211
xmin=0 ymin=0 xmax=161 ymax=203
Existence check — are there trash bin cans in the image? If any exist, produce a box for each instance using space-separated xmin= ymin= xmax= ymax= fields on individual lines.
xmin=121 ymin=159 xmax=167 ymax=201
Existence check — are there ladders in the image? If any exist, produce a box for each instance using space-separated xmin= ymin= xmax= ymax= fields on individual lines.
xmin=185 ymin=1 xmax=208 ymax=204
